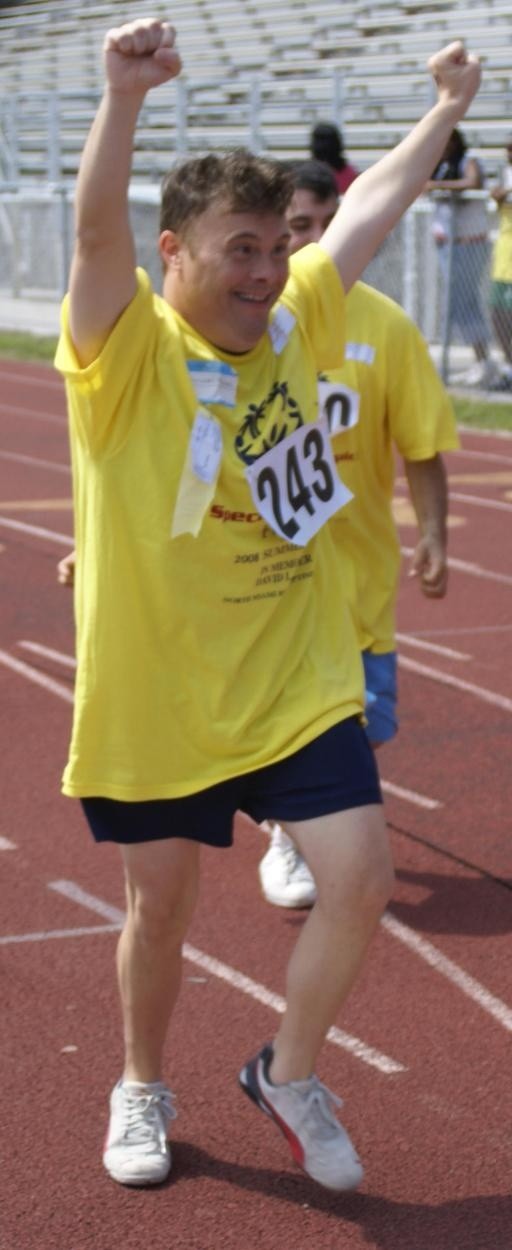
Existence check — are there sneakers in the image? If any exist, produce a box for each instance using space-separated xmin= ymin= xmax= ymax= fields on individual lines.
xmin=445 ymin=359 xmax=511 ymax=392
xmin=255 ymin=824 xmax=321 ymax=911
xmin=235 ymin=1040 xmax=364 ymax=1195
xmin=103 ymin=1072 xmax=174 ymax=1190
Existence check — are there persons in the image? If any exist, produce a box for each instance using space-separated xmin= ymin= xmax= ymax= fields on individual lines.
xmin=308 ymin=121 xmax=358 ymax=194
xmin=40 ymin=13 xmax=490 ymax=1193
xmin=425 ymin=127 xmax=491 ymax=389
xmin=488 ymin=143 xmax=512 ymax=390
xmin=48 ymin=153 xmax=468 ymax=914
